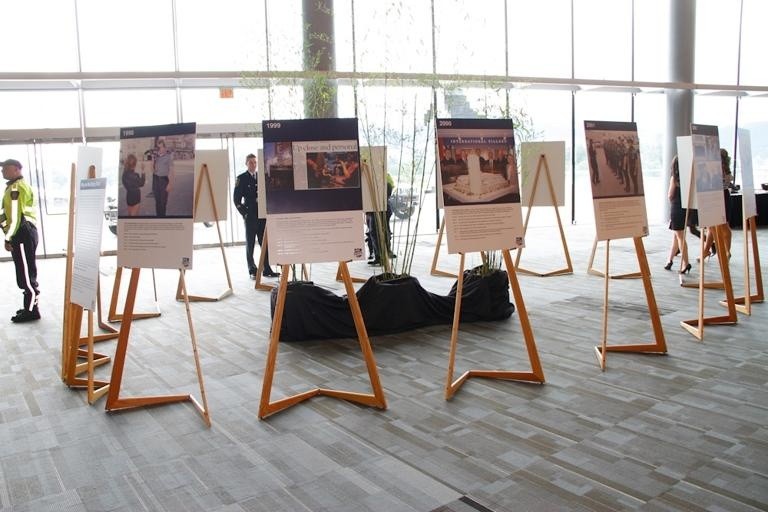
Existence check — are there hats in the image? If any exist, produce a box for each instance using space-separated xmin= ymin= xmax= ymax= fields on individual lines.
xmin=0 ymin=159 xmax=23 ymax=169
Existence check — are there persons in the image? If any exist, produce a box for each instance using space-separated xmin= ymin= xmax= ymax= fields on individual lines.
xmin=150 ymin=139 xmax=173 ymax=217
xmin=587 ymin=135 xmax=639 ymax=197
xmin=233 ymin=154 xmax=280 ymax=280
xmin=363 ymin=171 xmax=396 ymax=266
xmin=696 ymin=147 xmax=734 ymax=265
xmin=0 ymin=158 xmax=42 ymax=323
xmin=120 ymin=153 xmax=146 ymax=217
xmin=306 ymin=149 xmax=358 ymax=189
xmin=440 ymin=146 xmax=514 ymax=185
xmin=661 ymin=153 xmax=691 ymax=274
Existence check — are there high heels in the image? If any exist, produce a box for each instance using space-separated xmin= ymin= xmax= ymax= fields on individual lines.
xmin=681 ymin=264 xmax=691 ymax=274
xmin=665 ymin=260 xmax=672 ymax=270
xmin=697 ymin=251 xmax=711 ymax=263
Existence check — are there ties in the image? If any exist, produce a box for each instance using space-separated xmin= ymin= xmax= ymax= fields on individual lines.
xmin=252 ymin=174 xmax=254 ymax=178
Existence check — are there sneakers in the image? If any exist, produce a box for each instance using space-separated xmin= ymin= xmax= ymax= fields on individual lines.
xmin=12 ymin=309 xmax=40 ymax=323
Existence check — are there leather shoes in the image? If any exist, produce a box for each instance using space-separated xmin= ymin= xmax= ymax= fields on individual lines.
xmin=711 ymin=244 xmax=716 ymax=257
xmin=368 ymin=260 xmax=381 ymax=264
xmin=251 ymin=273 xmax=256 ymax=280
xmin=263 ymin=272 xmax=280 ymax=276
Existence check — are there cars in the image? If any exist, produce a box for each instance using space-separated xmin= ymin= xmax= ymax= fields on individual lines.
xmin=392 ymin=184 xmax=419 ymax=204
xmin=106 ymin=182 xmax=215 ymax=235
